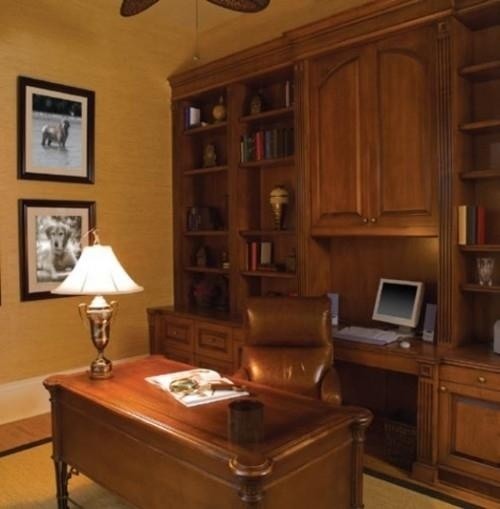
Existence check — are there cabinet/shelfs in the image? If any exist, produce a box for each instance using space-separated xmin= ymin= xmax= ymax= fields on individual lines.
xmin=309 ymin=24 xmax=441 ymax=239
xmin=437 ymin=360 xmax=500 ymax=488
xmin=171 ymin=60 xmax=303 ymax=321
xmin=145 ymin=306 xmax=245 ymax=378
xmin=449 ymin=0 xmax=500 ymax=294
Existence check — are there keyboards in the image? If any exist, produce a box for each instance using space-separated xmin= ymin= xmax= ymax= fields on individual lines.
xmin=331 ymin=324 xmax=399 ymax=346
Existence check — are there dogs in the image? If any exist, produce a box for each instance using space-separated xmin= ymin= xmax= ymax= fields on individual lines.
xmin=37 ymin=223 xmax=78 ymax=281
xmin=41 ymin=119 xmax=69 ymax=149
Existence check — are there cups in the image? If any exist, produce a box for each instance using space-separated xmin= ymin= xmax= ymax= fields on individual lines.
xmin=477 ymin=257 xmax=495 ymax=287
xmin=227 ymin=400 xmax=266 ymax=444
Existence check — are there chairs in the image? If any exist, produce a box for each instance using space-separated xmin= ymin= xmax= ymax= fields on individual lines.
xmin=236 ymin=294 xmax=343 ymax=406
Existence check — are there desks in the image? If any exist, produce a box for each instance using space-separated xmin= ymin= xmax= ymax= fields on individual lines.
xmin=43 ymin=354 xmax=375 ymax=509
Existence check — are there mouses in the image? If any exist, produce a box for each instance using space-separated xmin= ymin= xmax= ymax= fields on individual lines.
xmin=400 ymin=339 xmax=413 ymax=349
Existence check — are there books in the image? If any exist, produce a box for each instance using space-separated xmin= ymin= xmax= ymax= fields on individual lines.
xmin=457 ymin=204 xmax=489 ymax=247
xmin=183 ymin=107 xmax=202 ymax=131
xmin=239 ymin=126 xmax=293 ymax=163
xmin=144 ymin=367 xmax=249 ymax=409
xmin=246 ymin=239 xmax=273 ymax=272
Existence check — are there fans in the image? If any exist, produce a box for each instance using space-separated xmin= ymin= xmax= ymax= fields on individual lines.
xmin=119 ymin=0 xmax=271 ymax=61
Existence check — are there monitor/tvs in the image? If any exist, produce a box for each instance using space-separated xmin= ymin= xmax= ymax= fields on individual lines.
xmin=372 ymin=277 xmax=425 ymax=337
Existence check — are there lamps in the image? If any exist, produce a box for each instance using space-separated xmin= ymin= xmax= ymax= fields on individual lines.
xmin=50 ymin=243 xmax=145 ymax=381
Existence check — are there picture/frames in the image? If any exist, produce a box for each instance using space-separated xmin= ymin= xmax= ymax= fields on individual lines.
xmin=18 ymin=198 xmax=97 ymax=302
xmin=16 ymin=75 xmax=95 ymax=184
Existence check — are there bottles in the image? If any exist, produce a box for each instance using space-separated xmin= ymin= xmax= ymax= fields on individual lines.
xmin=188 ymin=207 xmax=200 ymax=231
xmin=281 ymin=79 xmax=293 ymax=108
xmin=250 ymin=240 xmax=260 ymax=273
xmin=268 ymin=183 xmax=290 ymax=230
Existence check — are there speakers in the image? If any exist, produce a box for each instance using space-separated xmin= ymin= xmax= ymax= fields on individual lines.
xmin=423 ymin=304 xmax=436 ymax=343
xmin=325 ymin=292 xmax=341 ymax=327
